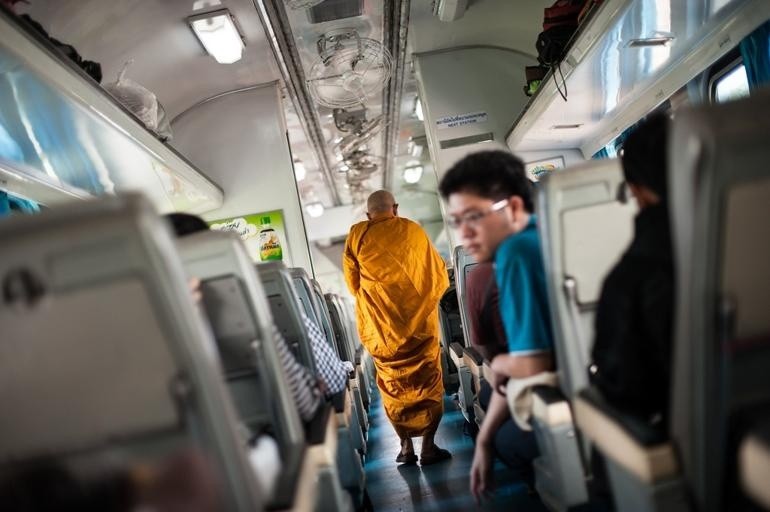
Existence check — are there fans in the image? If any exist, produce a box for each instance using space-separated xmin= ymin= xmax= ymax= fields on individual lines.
xmin=306 ymin=22 xmax=393 ymax=189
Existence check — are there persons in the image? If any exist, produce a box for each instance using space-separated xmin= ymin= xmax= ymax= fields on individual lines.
xmin=0 ymin=199 xmax=358 ymax=512
xmin=341 ymin=188 xmax=453 ymax=467
xmin=587 ymin=110 xmax=672 ymax=430
xmin=438 ymin=148 xmax=559 ymax=511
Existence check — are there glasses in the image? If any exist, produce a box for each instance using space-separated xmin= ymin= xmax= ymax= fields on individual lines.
xmin=446 ymin=199 xmax=509 ymax=229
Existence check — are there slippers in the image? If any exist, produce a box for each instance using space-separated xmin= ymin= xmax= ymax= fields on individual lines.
xmin=396 ymin=450 xmax=418 ymax=463
xmin=420 ymin=449 xmax=452 ymax=466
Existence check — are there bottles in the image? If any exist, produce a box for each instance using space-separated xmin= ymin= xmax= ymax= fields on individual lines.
xmin=258 ymin=217 xmax=282 ymax=263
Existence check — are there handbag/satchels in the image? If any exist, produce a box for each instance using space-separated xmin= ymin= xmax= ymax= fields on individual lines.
xmin=536 ymin=0 xmax=591 ymax=72
